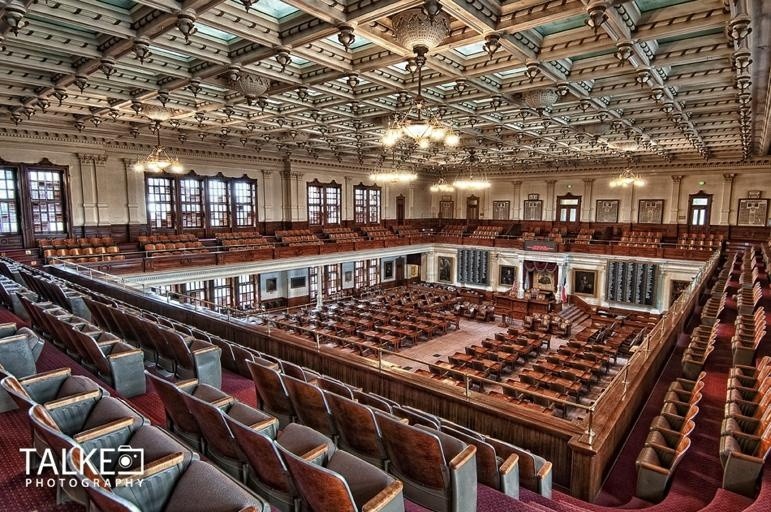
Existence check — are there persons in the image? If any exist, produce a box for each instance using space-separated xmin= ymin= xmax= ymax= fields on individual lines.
xmin=503 ymin=269 xmax=513 ymax=281
xmin=439 ymin=259 xmax=450 ymax=280
xmin=582 ymin=275 xmax=592 ymax=289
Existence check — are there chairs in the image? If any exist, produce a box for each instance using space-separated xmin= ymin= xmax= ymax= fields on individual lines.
xmin=0 ymin=217 xmax=771 ymax=510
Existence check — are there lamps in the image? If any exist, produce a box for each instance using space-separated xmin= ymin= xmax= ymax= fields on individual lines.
xmin=361 ymin=141 xmax=497 ymax=199
xmin=124 ymin=102 xmax=191 ymax=175
xmin=375 ymin=6 xmax=463 ymax=153
xmin=605 ymin=148 xmax=647 ymax=189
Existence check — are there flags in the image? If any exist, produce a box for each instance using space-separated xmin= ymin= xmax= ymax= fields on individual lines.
xmin=562 ymin=277 xmax=568 ymax=303
xmin=510 ymin=272 xmax=518 ymax=292
